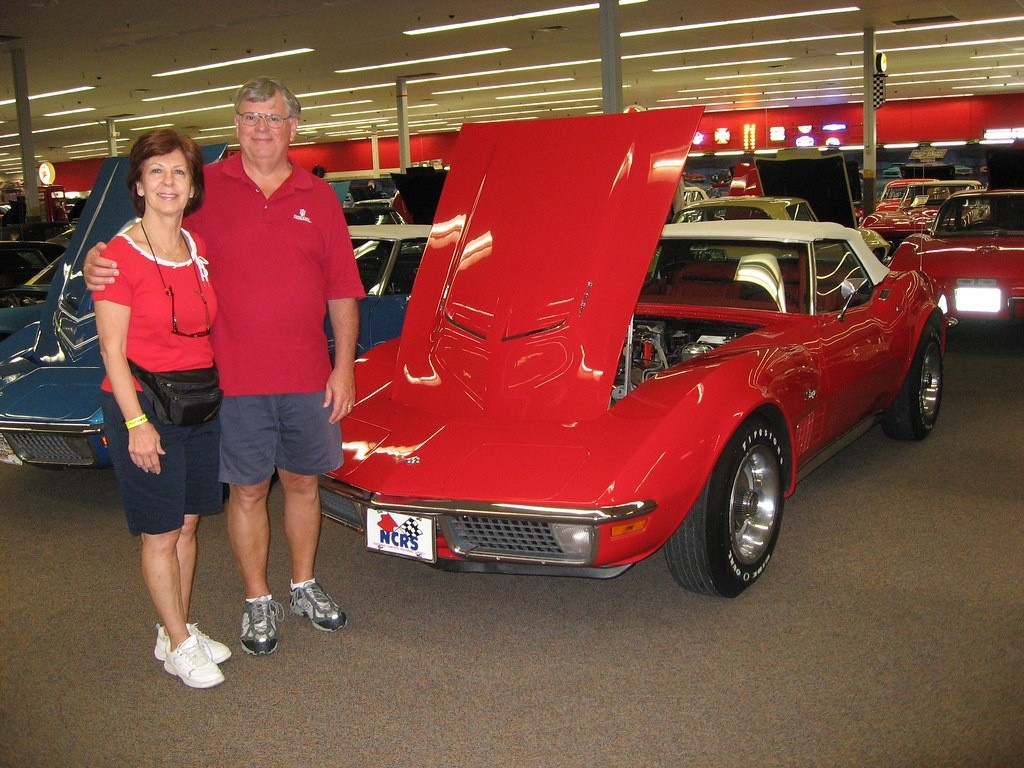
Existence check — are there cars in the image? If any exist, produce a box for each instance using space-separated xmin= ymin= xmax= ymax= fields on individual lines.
xmin=885 ymin=188 xmax=1024 ymax=327
xmin=1 ymin=143 xmax=448 ymax=470
xmin=315 ymin=105 xmax=960 ymax=600
xmin=646 ymin=147 xmax=891 ymax=277
xmin=665 ymin=163 xmax=994 ymax=257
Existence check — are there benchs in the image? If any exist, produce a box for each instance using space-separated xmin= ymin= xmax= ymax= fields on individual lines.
xmin=671 ymin=261 xmax=849 ymax=314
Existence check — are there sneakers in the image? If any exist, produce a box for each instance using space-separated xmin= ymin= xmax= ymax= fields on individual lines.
xmin=239 ymin=596 xmax=285 ymax=655
xmin=155 ymin=620 xmax=232 ymax=666
xmin=164 ymin=633 xmax=226 ymax=689
xmin=289 ymin=580 xmax=347 ymax=632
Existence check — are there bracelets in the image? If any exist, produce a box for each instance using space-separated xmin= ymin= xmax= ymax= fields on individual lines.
xmin=124 ymin=414 xmax=148 ymax=430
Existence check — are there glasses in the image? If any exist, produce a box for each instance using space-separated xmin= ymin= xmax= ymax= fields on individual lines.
xmin=238 ymin=109 xmax=294 ymax=129
xmin=167 ymin=284 xmax=210 ymax=336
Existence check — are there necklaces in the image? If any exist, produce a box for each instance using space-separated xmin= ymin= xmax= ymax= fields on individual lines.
xmin=141 ymin=221 xmax=184 ymax=270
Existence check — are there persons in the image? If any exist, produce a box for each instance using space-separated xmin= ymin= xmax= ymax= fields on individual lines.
xmin=344 ymin=180 xmax=377 ymax=205
xmin=84 ymin=75 xmax=367 ymax=655
xmin=91 ymin=128 xmax=231 ymax=689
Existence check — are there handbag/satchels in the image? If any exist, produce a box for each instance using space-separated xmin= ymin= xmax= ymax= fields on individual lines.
xmin=125 ymin=356 xmax=224 ymax=428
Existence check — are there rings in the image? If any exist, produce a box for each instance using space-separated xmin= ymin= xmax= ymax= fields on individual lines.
xmin=348 ymin=405 xmax=352 ymax=407
